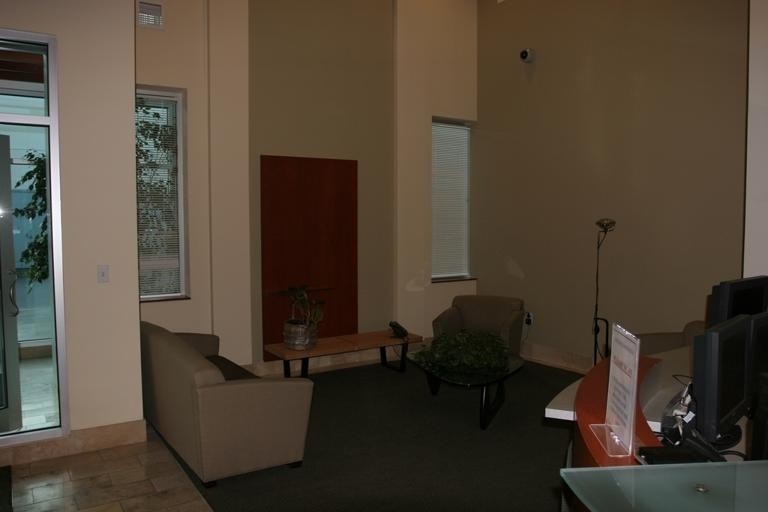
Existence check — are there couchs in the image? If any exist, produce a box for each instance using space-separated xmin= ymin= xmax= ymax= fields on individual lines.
xmin=145 ymin=322 xmax=315 ymax=487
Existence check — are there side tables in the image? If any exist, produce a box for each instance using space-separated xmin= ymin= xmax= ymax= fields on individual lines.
xmin=406 ymin=346 xmax=524 ymax=430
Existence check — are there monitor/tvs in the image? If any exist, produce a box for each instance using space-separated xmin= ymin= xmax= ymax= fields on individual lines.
xmin=704 ymin=275 xmax=768 ymax=328
xmin=692 ymin=313 xmax=753 ymax=450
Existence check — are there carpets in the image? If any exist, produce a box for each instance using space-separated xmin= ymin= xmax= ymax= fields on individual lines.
xmin=158 ymin=357 xmax=559 ymax=510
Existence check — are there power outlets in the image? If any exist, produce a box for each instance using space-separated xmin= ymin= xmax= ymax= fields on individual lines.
xmin=524 ymin=313 xmax=534 ymax=327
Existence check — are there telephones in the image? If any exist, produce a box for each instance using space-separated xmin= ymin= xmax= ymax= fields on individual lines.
xmin=388 ymin=320 xmax=409 ymax=339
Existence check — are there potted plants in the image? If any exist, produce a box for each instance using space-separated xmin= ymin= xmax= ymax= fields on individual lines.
xmin=282 ymin=283 xmax=325 ymax=352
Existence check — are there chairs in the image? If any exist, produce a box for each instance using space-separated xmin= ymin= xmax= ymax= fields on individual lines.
xmin=432 ymin=295 xmax=524 ymax=355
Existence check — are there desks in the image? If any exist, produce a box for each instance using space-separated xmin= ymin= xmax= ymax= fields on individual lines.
xmin=544 ymin=345 xmax=748 ymax=467
xmin=560 ymin=460 xmax=768 ymax=512
